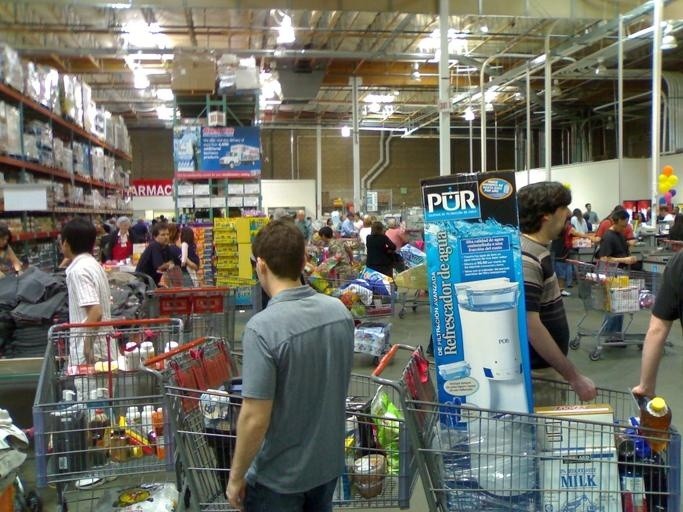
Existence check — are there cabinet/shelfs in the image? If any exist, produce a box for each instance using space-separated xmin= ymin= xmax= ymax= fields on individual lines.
xmin=0 ymin=81 xmax=133 ymax=274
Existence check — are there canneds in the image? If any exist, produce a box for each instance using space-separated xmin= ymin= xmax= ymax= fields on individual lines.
xmin=616 ymin=435 xmax=635 ymax=456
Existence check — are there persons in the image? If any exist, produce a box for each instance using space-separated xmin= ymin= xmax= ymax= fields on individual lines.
xmin=626 ymin=201 xmax=682 ymax=241
xmin=517 ymin=182 xmax=596 ymax=407
xmin=134 ymin=223 xmax=169 ymax=288
xmin=90 ymin=215 xmax=132 ymax=265
xmin=270 ymin=201 xmax=372 ymax=264
xmin=583 ymin=203 xmax=601 ymax=226
xmin=0 ymin=226 xmax=23 ymax=278
xmin=367 ymin=223 xmax=396 ymax=277
xmin=551 ymin=218 xmax=589 ymax=297
xmin=133 ymin=218 xmax=148 ymax=243
xmin=633 ymin=246 xmax=683 ymax=398
xmin=63 ymin=218 xmax=120 ymax=489
xmin=571 ymin=208 xmax=588 ymax=234
xmin=383 ymin=217 xmax=409 ymax=256
xmin=169 ymin=222 xmax=192 ymax=288
xmin=597 ymin=206 xmax=637 ymax=348
xmin=149 ymin=215 xmax=168 ymax=227
xmin=180 ymin=228 xmax=200 ymax=289
xmin=226 ymin=218 xmax=356 ymax=511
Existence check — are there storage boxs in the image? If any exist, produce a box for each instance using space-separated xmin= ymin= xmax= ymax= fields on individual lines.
xmin=571 ymin=236 xmax=592 ymax=248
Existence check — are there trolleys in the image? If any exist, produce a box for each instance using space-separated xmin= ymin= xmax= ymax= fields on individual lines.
xmin=308 ymin=277 xmax=394 ymax=366
xmin=33 ymin=317 xmax=184 ymax=512
xmin=565 ymin=259 xmax=673 ymax=361
xmin=371 ymin=344 xmax=683 ymax=511
xmin=393 ymin=267 xmax=430 ymax=319
xmin=145 ymin=285 xmax=238 ymax=352
xmin=139 ymin=337 xmax=409 ymax=512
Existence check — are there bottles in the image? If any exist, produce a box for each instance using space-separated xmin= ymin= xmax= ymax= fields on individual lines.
xmin=164 ymin=342 xmax=178 ymax=368
xmin=142 ymin=406 xmax=155 ymax=434
xmin=90 ymin=409 xmax=110 ymax=466
xmin=50 ymin=390 xmax=88 ymax=475
xmin=124 ymin=342 xmax=139 ymax=370
xmin=153 ymin=408 xmax=165 ymax=457
xmin=140 ymin=342 xmax=154 ymax=362
xmin=126 ymin=407 xmax=140 ymax=419
xmin=109 ymin=430 xmax=129 ymax=463
xmin=94 ymin=388 xmax=111 ymax=420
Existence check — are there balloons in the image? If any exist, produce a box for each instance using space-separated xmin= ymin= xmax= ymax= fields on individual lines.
xmin=657 ymin=177 xmax=677 ymax=206
xmin=662 ymin=166 xmax=673 ymax=176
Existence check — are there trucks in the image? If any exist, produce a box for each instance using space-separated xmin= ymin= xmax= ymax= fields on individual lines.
xmin=219 ymin=145 xmax=260 ymax=169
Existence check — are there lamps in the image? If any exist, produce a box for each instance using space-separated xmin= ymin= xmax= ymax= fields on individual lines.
xmin=551 ymin=79 xmax=562 ymax=97
xmin=472 ymin=17 xmax=489 ymax=35
xmin=515 ymin=89 xmax=526 ymax=100
xmin=410 ymin=62 xmax=421 ymax=81
xmin=465 ymin=109 xmax=475 ymax=121
xmin=660 ymin=25 xmax=678 ymax=50
xmin=485 ymin=101 xmax=494 ymax=112
xmin=595 ymin=57 xmax=608 ymax=76
